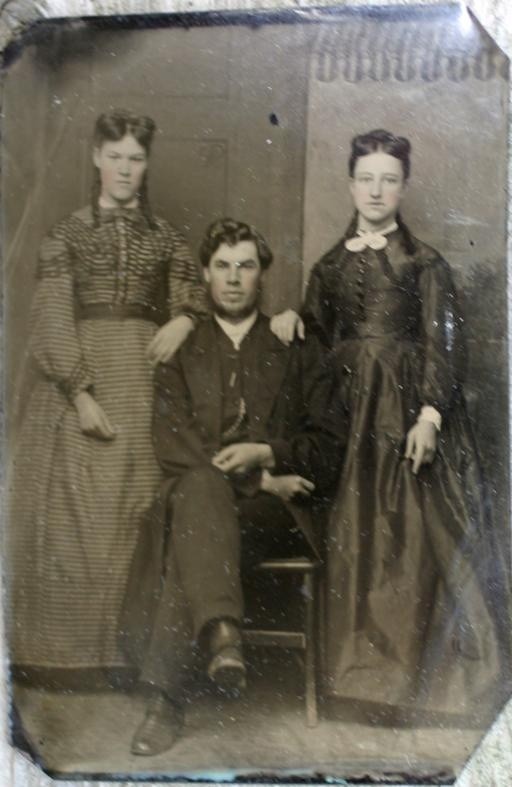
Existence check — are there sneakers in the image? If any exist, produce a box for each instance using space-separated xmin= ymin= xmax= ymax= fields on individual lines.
xmin=205 ymin=622 xmax=264 ymax=702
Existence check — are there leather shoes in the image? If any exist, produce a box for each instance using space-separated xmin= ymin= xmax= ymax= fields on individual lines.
xmin=130 ymin=689 xmax=185 ymax=756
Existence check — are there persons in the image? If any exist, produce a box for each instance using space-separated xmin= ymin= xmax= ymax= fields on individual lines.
xmin=0 ymin=109 xmax=212 ymax=702
xmin=109 ymin=216 xmax=320 ymax=765
xmin=267 ymin=128 xmax=509 ymax=733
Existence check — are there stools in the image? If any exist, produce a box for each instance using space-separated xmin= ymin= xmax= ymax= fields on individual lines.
xmin=183 ymin=556 xmax=320 ymax=726
